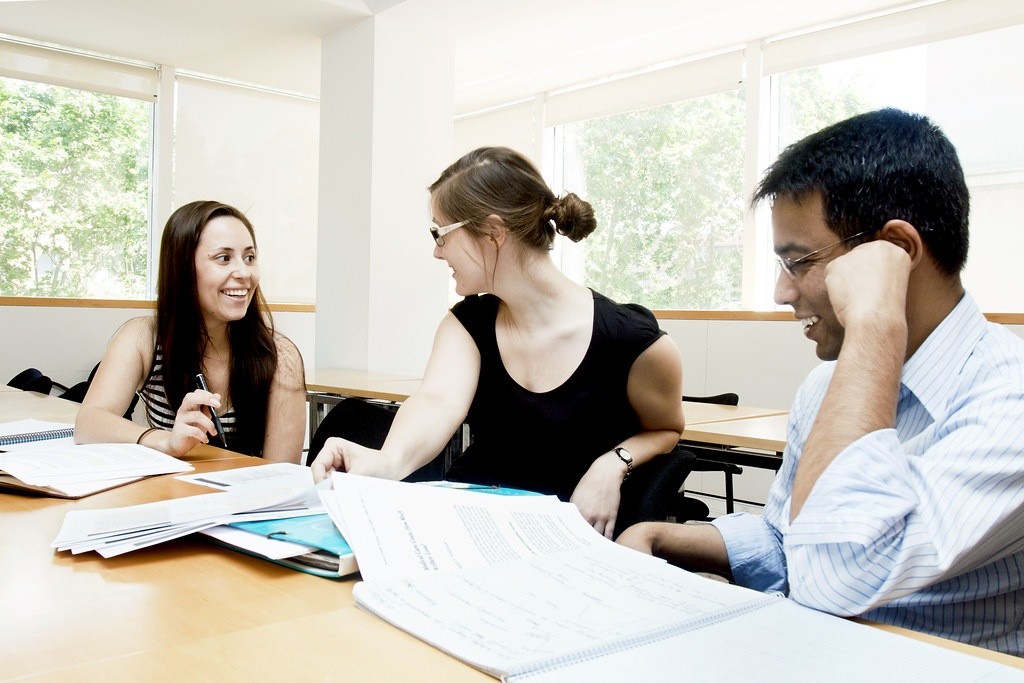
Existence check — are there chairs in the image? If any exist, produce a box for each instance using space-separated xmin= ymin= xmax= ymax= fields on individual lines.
xmin=307 ymin=397 xmax=461 ymax=484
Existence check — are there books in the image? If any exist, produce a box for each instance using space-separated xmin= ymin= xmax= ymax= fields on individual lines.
xmin=352 ymin=563 xmax=1024 ymax=683
xmin=0 ymin=418 xmax=195 ymax=500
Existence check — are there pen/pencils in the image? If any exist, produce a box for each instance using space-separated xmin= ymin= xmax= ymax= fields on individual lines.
xmin=196 ymin=373 xmax=227 ymax=448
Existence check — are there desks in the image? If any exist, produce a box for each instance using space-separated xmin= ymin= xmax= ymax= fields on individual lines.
xmin=305 ymin=367 xmax=420 ymax=401
xmin=682 ymin=399 xmax=791 ymax=518
xmin=0 ymin=382 xmax=1023 ymax=683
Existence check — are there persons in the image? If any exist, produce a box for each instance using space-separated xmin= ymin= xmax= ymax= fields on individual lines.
xmin=311 ymin=146 xmax=685 ymax=542
xmin=614 ymin=108 xmax=1023 ymax=659
xmin=73 ymin=200 xmax=308 ymax=465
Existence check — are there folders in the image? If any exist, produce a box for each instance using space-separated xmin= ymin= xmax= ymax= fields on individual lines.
xmin=221 ymin=481 xmax=544 ymax=579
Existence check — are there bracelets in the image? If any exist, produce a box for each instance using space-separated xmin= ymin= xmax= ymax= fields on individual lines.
xmin=137 ymin=427 xmax=164 ymax=444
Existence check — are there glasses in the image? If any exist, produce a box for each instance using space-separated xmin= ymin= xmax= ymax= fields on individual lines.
xmin=430 ymin=214 xmax=485 ymax=248
xmin=774 ymin=224 xmax=935 ymax=282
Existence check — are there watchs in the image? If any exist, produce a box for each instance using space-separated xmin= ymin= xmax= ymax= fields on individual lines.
xmin=611 ymin=447 xmax=633 ymax=480
